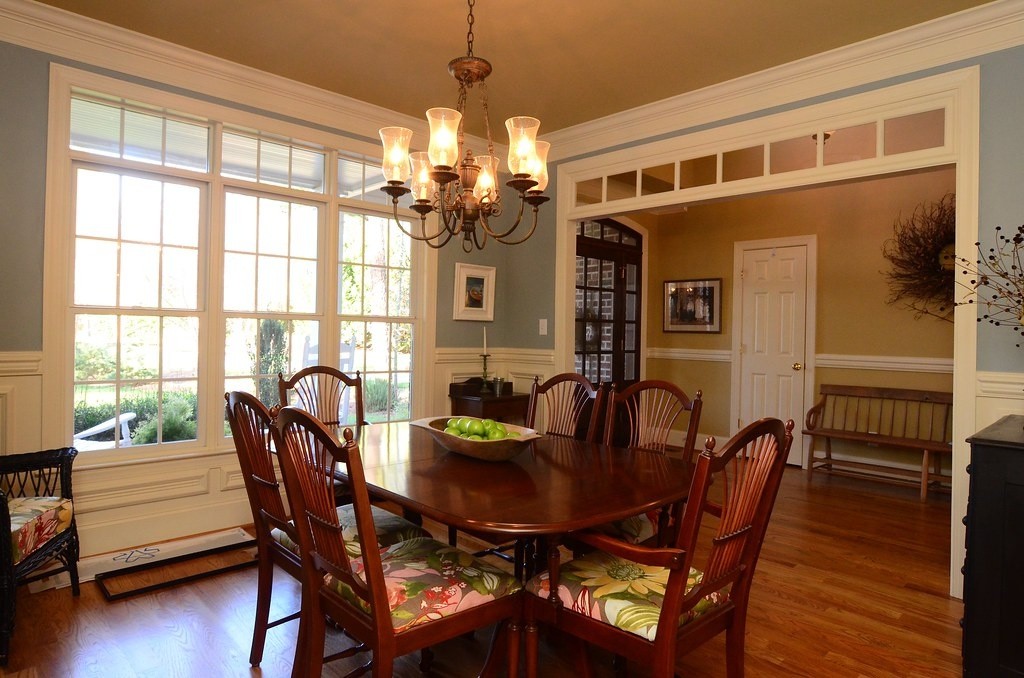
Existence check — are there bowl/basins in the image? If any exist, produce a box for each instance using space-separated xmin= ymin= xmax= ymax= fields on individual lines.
xmin=409 ymin=416 xmax=542 ymax=461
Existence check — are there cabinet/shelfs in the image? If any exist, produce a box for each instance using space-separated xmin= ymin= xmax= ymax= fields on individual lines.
xmin=960 ymin=413 xmax=1024 ymax=677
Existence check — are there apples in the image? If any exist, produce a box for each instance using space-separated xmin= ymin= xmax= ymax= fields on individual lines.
xmin=443 ymin=417 xmax=520 ymax=441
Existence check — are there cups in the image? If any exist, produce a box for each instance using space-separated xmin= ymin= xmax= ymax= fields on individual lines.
xmin=493 ymin=377 xmax=504 ymax=395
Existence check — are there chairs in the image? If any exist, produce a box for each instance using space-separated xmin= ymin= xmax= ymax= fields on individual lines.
xmin=224 ymin=366 xmax=794 ymax=678
xmin=0 ymin=446 xmax=81 ymax=651
xmin=801 ymin=383 xmax=952 ymax=505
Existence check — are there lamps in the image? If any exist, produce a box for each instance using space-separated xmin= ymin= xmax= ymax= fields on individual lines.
xmin=379 ymin=0 xmax=551 ymax=253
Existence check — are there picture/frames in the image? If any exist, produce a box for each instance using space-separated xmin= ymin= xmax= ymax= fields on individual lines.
xmin=662 ymin=277 xmax=723 ymax=333
xmin=453 ymin=261 xmax=497 ymax=322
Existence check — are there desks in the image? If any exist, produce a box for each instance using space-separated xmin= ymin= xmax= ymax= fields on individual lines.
xmin=449 ymin=391 xmax=530 ymax=427
xmin=258 ymin=418 xmax=714 ymax=678
